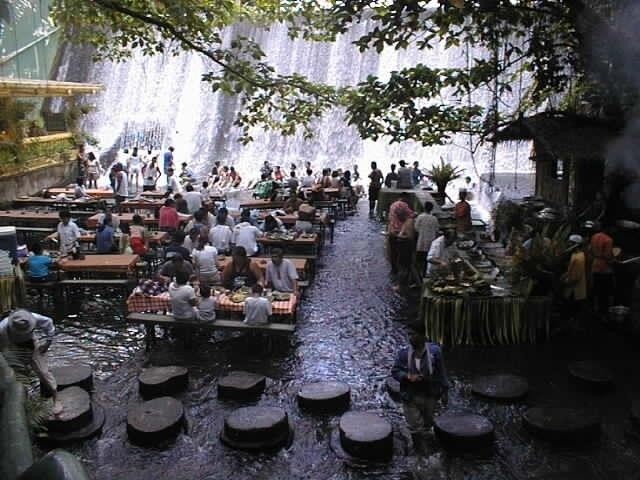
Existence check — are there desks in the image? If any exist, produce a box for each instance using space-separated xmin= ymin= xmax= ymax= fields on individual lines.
xmin=372 ymin=180 xmax=639 ymax=347
xmin=129 ymin=283 xmax=297 ymax=339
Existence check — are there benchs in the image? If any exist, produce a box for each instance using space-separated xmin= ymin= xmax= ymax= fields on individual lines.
xmin=126 ymin=313 xmax=296 ymax=354
xmin=0 ymin=172 xmax=349 ymax=297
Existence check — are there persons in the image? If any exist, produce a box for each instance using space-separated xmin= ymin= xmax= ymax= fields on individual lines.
xmin=522 ymin=228 xmax=550 ymax=261
xmin=368 ymin=161 xmax=473 ymax=288
xmin=588 ymin=219 xmax=621 ymax=325
xmin=76 ymin=144 xmax=367 ymax=258
xmin=48 ymin=211 xmax=81 ymax=257
xmin=392 ymin=322 xmax=443 ymax=455
xmin=1 ymin=308 xmax=56 ymax=369
xmin=156 ymin=228 xmax=298 ymax=341
xmin=24 ymin=244 xmax=50 ymax=283
xmin=561 ymin=235 xmax=586 ymax=336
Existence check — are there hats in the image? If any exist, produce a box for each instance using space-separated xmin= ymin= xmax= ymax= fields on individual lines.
xmin=8 ymin=310 xmax=36 ymax=335
